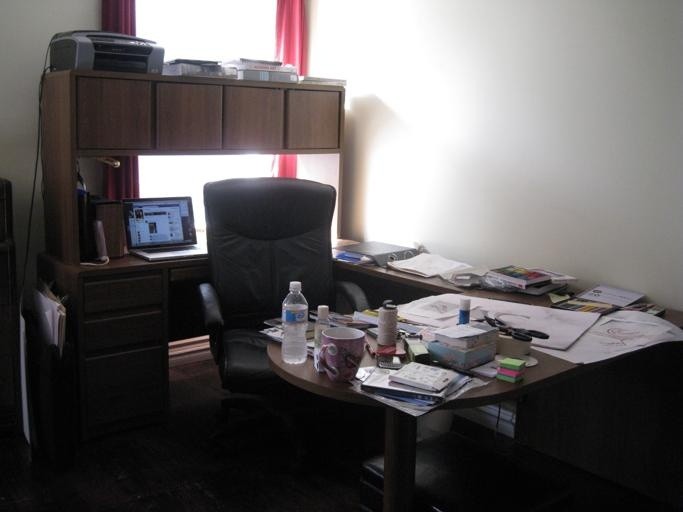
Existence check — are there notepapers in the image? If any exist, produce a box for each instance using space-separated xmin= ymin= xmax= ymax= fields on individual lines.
xmin=495 ymin=357 xmax=527 ymax=384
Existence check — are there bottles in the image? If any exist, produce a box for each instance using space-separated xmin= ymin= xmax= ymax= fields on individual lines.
xmin=281 ymin=280 xmax=309 ymax=365
xmin=312 ymin=304 xmax=329 ymax=371
xmin=459 ymin=297 xmax=470 ymax=325
xmin=378 ymin=299 xmax=398 ymax=346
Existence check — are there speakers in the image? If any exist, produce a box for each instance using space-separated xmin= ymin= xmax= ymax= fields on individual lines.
xmin=88 ymin=196 xmax=125 ymax=259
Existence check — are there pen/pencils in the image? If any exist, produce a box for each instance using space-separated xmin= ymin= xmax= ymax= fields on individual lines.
xmin=432 ymin=360 xmax=478 ymax=378
xmin=366 ymin=341 xmax=376 ymax=356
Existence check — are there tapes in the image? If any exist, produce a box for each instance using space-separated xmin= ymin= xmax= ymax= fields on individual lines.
xmin=496 ymin=333 xmax=532 ymax=355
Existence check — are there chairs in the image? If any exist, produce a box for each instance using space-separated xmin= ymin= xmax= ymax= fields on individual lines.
xmin=199 ymin=178 xmax=370 ymax=467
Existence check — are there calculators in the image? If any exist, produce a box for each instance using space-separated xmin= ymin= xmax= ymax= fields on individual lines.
xmin=377 ymin=356 xmax=402 ymax=369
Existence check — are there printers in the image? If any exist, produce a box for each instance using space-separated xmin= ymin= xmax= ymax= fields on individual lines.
xmin=49 ymin=29 xmax=165 ymax=74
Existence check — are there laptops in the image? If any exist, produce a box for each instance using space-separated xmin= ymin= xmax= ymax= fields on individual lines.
xmin=120 ymin=196 xmax=208 ymax=263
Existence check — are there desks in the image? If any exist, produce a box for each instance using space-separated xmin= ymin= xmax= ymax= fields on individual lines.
xmin=265 ymin=250 xmax=683 ymax=512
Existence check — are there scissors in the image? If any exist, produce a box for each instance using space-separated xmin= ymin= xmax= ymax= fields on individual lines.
xmin=485 ymin=316 xmax=549 ymax=341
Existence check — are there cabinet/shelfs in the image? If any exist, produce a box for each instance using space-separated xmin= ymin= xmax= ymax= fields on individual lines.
xmin=37 ymin=68 xmax=347 ymax=444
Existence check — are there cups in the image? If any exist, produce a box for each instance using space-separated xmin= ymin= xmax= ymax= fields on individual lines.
xmin=318 ymin=328 xmax=366 ymax=383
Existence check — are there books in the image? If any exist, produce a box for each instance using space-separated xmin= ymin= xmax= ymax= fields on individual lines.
xmin=484 ymin=277 xmax=567 ymax=296
xmin=160 ymin=58 xmax=347 ymax=88
xmin=487 ymin=275 xmax=552 ymax=289
xmin=259 ymin=311 xmax=371 ymax=358
xmin=551 ymin=297 xmax=619 ymax=315
xmin=488 ymin=264 xmax=551 ymax=283
xmin=359 ymin=365 xmax=449 ymax=401
